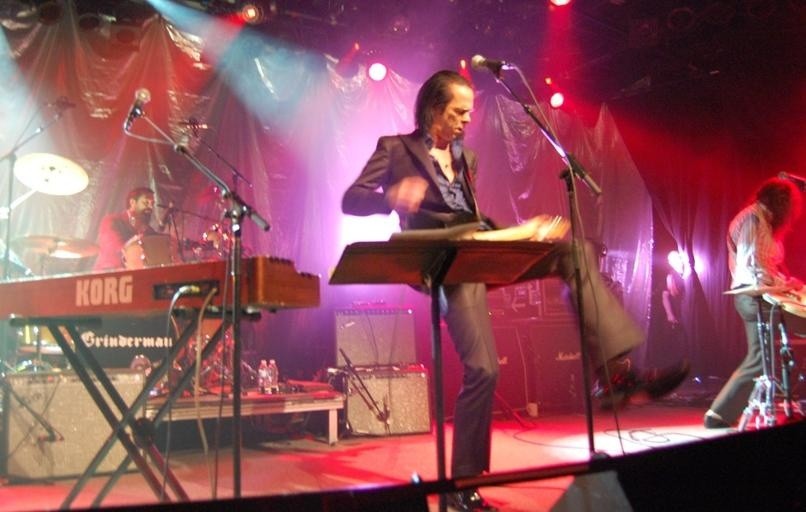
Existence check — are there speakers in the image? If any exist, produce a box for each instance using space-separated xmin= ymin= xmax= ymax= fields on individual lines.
xmin=334 ymin=307 xmax=417 ymax=369
xmin=3 ymin=370 xmax=148 ymax=482
xmin=424 ymin=469 xmax=634 ymax=512
xmin=345 ymin=368 xmax=432 ymax=436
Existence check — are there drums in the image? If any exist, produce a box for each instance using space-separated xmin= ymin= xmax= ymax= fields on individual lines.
xmin=121 ymin=231 xmax=174 ymax=269
xmin=202 ymin=223 xmax=230 ymax=251
xmin=169 ymin=309 xmax=234 ymax=394
xmin=18 ymin=323 xmax=77 ymax=351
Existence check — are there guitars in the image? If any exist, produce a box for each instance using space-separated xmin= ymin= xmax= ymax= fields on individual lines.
xmin=763 ymin=277 xmax=806 ymax=318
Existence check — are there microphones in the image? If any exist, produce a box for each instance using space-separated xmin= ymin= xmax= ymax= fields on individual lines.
xmin=779 ymin=172 xmax=806 ymax=191
xmin=473 ymin=54 xmax=505 ymax=84
xmin=122 ymin=87 xmax=153 ymax=130
xmin=383 ymin=393 xmax=391 ymax=426
xmin=177 ymin=121 xmax=210 ymax=132
xmin=157 ymin=200 xmax=173 ymax=229
xmin=45 ymin=98 xmax=82 ymax=111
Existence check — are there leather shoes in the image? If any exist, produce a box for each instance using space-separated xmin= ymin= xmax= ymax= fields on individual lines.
xmin=593 ymin=358 xmax=689 ymax=411
xmin=702 ymin=411 xmax=734 ymax=428
xmin=445 ymin=488 xmax=498 ymax=512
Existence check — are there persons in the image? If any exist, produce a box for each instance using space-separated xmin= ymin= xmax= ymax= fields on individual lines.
xmin=90 ymin=188 xmax=163 ymax=271
xmin=342 ymin=69 xmax=690 ymax=512
xmin=697 ymin=176 xmax=802 ymax=428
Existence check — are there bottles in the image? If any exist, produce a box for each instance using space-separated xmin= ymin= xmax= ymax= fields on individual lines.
xmin=131 ymin=349 xmax=156 ymax=401
xmin=258 ymin=358 xmax=279 ymax=395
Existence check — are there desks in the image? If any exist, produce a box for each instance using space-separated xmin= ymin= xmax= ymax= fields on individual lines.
xmin=146 ymin=379 xmax=344 ymax=445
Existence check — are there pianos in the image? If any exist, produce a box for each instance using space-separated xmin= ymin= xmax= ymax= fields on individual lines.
xmin=0 ymin=254 xmax=320 ymax=327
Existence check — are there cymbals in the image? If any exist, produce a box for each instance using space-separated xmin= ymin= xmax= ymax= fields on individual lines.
xmin=13 ymin=152 xmax=89 ymax=195
xmin=10 ymin=233 xmax=100 ymax=256
